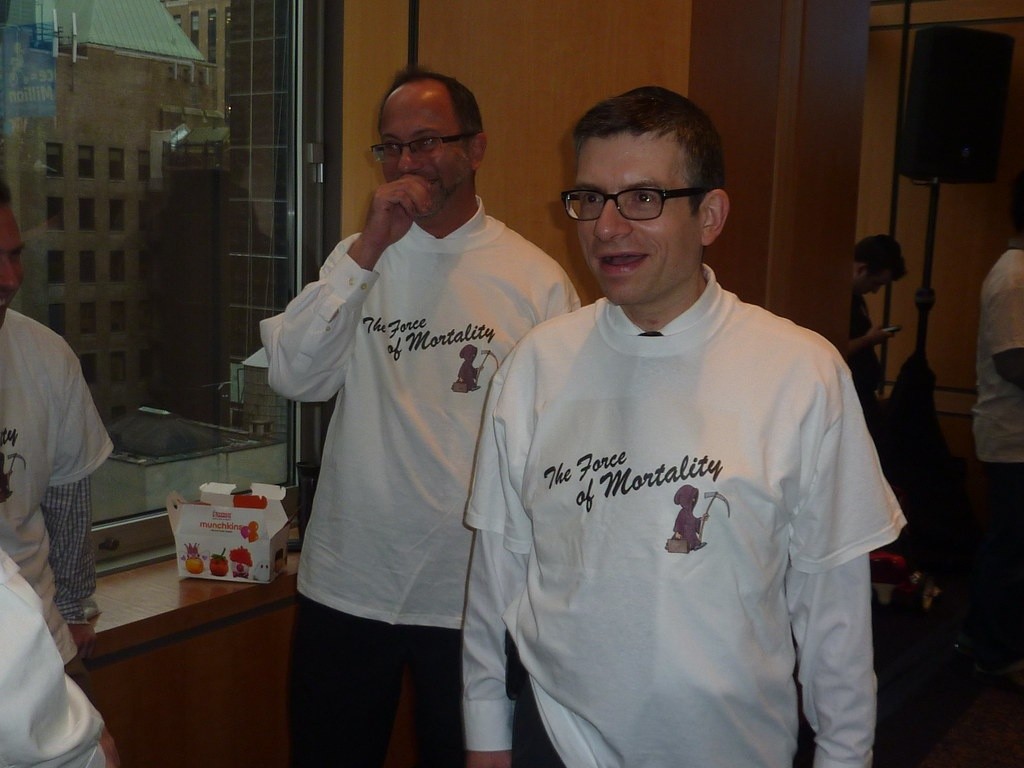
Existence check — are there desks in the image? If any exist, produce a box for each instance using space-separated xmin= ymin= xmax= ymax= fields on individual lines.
xmin=800 ymin=586 xmax=964 ymax=768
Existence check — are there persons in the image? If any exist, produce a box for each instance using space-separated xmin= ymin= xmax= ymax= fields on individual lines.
xmin=960 ymin=170 xmax=1024 ymax=674
xmin=0 ymin=182 xmax=121 ymax=768
xmin=259 ymin=65 xmax=580 ymax=768
xmin=463 ymin=85 xmax=906 ymax=768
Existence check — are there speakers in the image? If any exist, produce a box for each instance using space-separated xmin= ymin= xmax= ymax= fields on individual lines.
xmin=897 ymin=25 xmax=1016 ymax=182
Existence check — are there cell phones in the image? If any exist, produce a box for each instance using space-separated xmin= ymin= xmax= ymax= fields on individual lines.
xmin=881 ymin=325 xmax=901 ymax=332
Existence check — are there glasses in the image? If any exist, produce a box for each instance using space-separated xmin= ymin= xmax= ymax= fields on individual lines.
xmin=370 ymin=133 xmax=473 ymax=164
xmin=560 ymin=187 xmax=711 ymax=222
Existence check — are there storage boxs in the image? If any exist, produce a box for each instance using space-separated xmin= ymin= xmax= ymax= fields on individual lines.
xmin=165 ymin=482 xmax=302 ymax=584
